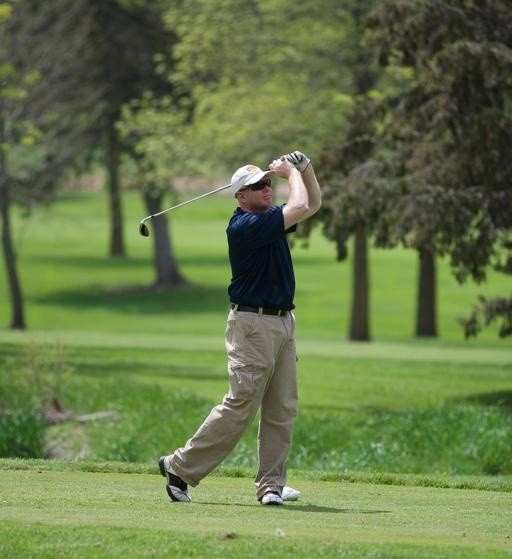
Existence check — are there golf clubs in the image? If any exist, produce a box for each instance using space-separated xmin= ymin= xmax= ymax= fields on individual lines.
xmin=140 ymin=167 xmax=271 ymax=235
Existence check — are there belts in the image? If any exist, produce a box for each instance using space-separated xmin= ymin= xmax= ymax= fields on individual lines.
xmin=231 ymin=303 xmax=285 ymax=316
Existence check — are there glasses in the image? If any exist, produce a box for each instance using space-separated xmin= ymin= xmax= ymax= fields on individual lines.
xmin=239 ymin=178 xmax=272 ymax=192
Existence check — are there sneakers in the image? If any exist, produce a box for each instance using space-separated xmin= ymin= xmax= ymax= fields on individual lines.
xmin=158 ymin=455 xmax=192 ymax=502
xmin=260 ymin=491 xmax=284 ymax=505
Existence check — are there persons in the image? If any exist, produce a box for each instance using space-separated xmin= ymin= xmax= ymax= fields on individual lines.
xmin=159 ymin=150 xmax=325 ymax=505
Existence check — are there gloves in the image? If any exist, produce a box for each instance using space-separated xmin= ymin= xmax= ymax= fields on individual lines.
xmin=281 ymin=151 xmax=311 ymax=173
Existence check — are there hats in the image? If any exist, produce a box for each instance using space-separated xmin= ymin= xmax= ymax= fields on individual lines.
xmin=230 ymin=164 xmax=277 ymax=198
